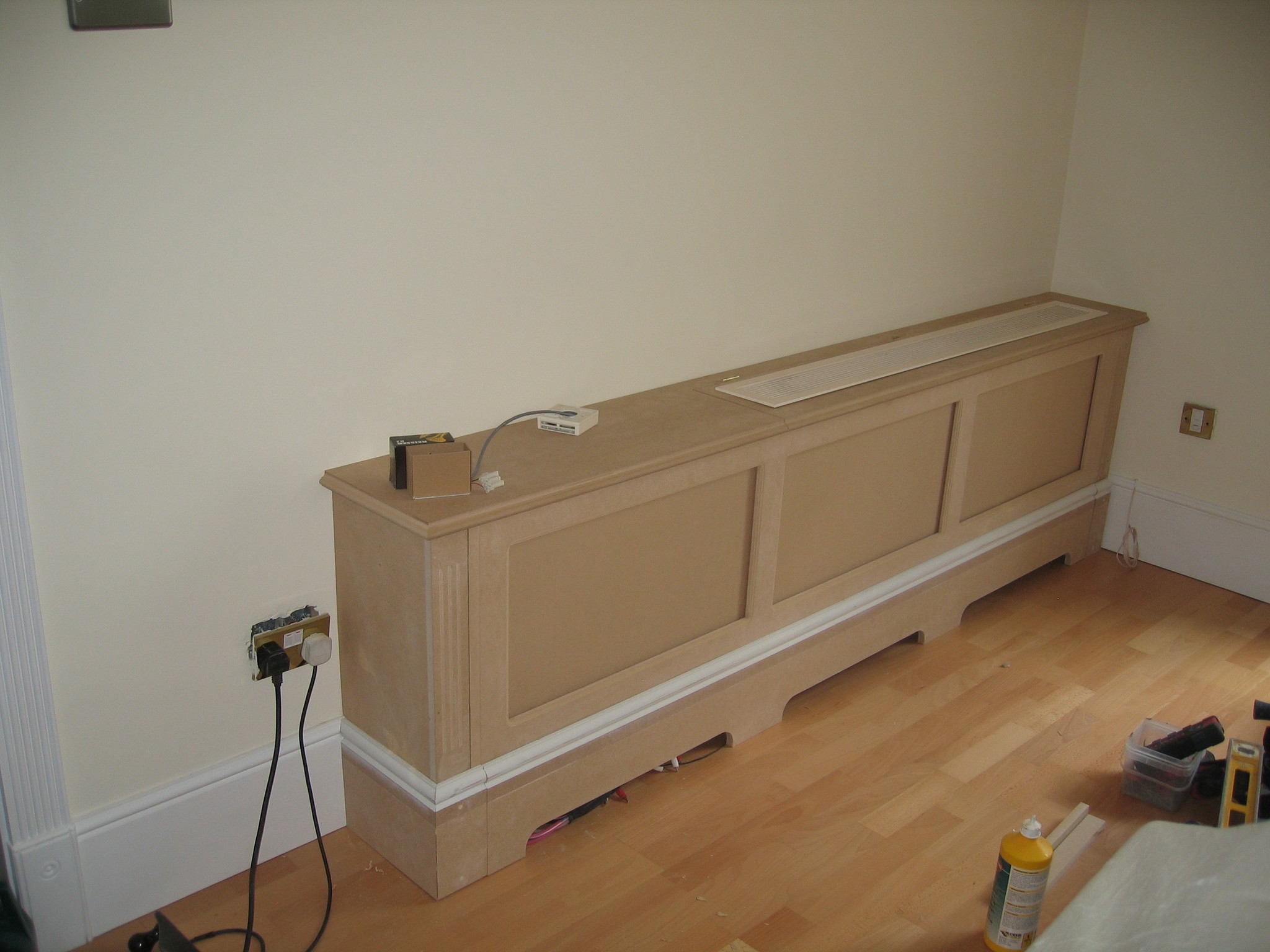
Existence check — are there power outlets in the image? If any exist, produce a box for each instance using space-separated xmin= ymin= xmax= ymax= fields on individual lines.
xmin=254 ymin=611 xmax=330 ymax=681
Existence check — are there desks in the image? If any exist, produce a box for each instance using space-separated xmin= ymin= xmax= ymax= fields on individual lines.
xmin=319 ymin=291 xmax=1149 ymax=901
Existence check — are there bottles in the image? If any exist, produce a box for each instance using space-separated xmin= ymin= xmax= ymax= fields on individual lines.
xmin=983 ymin=815 xmax=1053 ymax=952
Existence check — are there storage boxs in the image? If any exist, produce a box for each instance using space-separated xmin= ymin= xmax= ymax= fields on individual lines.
xmin=389 ymin=432 xmax=455 ymax=489
xmin=1120 ymin=718 xmax=1206 ymax=814
xmin=406 ymin=442 xmax=472 ymax=500
